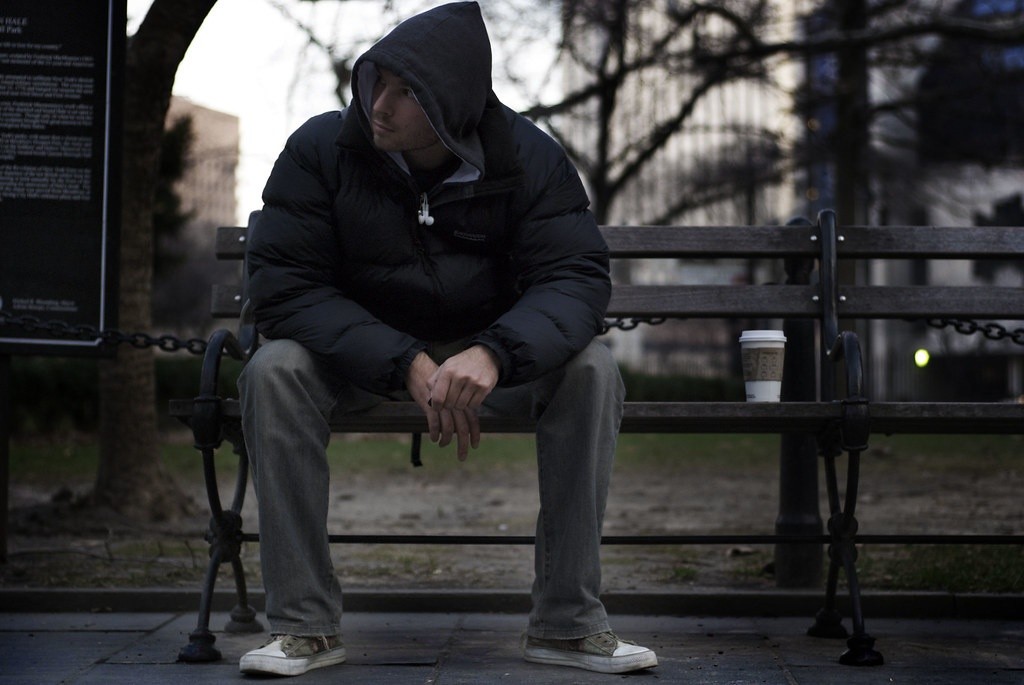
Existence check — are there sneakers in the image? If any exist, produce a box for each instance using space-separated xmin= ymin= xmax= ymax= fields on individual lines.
xmin=239 ymin=632 xmax=348 ymax=677
xmin=523 ymin=630 xmax=659 ymax=673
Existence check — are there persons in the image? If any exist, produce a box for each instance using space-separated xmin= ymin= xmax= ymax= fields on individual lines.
xmin=238 ymin=2 xmax=658 ymax=675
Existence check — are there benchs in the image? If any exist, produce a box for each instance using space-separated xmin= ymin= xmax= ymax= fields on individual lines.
xmin=167 ymin=210 xmax=1024 ymax=669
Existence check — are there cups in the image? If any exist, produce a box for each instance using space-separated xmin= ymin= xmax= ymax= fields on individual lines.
xmin=739 ymin=330 xmax=787 ymax=402
